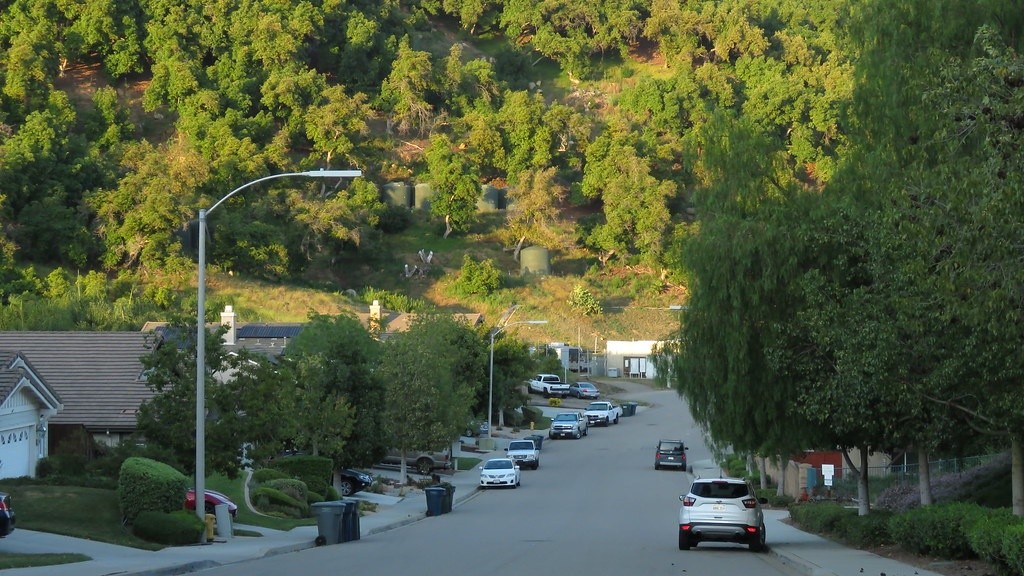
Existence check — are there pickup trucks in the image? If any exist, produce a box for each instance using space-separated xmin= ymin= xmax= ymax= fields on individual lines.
xmin=583 ymin=401 xmax=624 ymax=428
xmin=549 ymin=411 xmax=589 ymax=439
xmin=527 ymin=373 xmax=571 ymax=399
xmin=505 ymin=438 xmax=540 ymax=470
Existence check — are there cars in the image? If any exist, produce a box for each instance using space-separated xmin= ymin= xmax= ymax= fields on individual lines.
xmin=568 ymin=381 xmax=600 ymax=400
xmin=269 ymin=448 xmax=374 ymax=497
xmin=0 ymin=491 xmax=17 ymax=536
xmin=478 ymin=458 xmax=522 ymax=489
xmin=184 ymin=487 xmax=239 ymax=520
xmin=458 ymin=417 xmax=488 ymax=438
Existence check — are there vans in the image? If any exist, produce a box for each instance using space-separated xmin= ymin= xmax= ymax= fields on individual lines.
xmin=377 ymin=443 xmax=452 ymax=476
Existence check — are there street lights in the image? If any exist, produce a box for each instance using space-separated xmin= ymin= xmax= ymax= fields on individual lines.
xmin=487 ymin=320 xmax=548 ymax=439
xmin=193 ymin=167 xmax=364 ymax=544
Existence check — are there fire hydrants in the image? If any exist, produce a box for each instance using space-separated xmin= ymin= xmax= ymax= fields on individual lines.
xmin=205 ymin=514 xmax=216 ymax=543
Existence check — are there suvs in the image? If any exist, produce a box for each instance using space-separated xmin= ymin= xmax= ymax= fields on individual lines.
xmin=655 ymin=439 xmax=689 ymax=471
xmin=679 ymin=475 xmax=768 ymax=553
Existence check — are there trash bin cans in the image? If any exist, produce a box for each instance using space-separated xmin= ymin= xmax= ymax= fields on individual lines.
xmin=215 ymin=504 xmax=234 ymax=538
xmin=620 ymin=401 xmax=638 ymax=417
xmin=523 ymin=434 xmax=543 ymax=450
xmin=434 ymin=484 xmax=456 ymax=513
xmin=311 ymin=502 xmax=346 ymax=546
xmin=337 ymin=499 xmax=360 ymax=542
xmin=424 ymin=487 xmax=446 ymax=517
